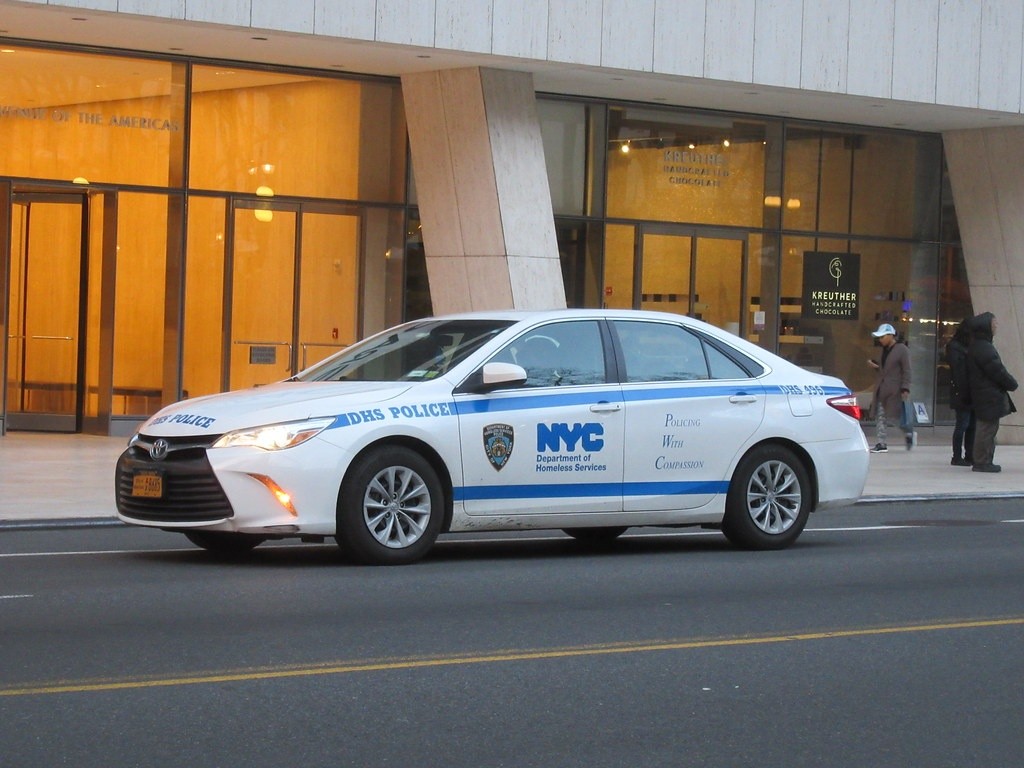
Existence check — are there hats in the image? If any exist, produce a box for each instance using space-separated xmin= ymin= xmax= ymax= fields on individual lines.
xmin=871 ymin=323 xmax=896 ymax=338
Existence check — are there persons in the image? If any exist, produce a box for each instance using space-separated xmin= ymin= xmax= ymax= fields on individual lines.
xmin=869 ymin=323 xmax=917 ymax=453
xmin=945 ymin=312 xmax=1019 ymax=473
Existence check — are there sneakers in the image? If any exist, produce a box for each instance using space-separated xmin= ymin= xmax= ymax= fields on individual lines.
xmin=870 ymin=443 xmax=888 ymax=453
xmin=905 ymin=431 xmax=917 ymax=451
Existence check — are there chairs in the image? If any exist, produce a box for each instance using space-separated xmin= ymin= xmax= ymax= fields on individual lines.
xmin=516 ymin=337 xmax=562 ymax=387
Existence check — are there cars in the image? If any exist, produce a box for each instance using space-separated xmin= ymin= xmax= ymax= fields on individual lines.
xmin=112 ymin=307 xmax=871 ymax=570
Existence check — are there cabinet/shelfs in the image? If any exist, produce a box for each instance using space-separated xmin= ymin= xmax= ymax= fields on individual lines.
xmin=748 ymin=303 xmax=825 ymax=373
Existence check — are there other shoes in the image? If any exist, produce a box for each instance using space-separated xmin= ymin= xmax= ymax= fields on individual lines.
xmin=964 ymin=458 xmax=974 ymax=464
xmin=972 ymin=464 xmax=1002 ymax=473
xmin=951 ymin=458 xmax=971 ymax=466
